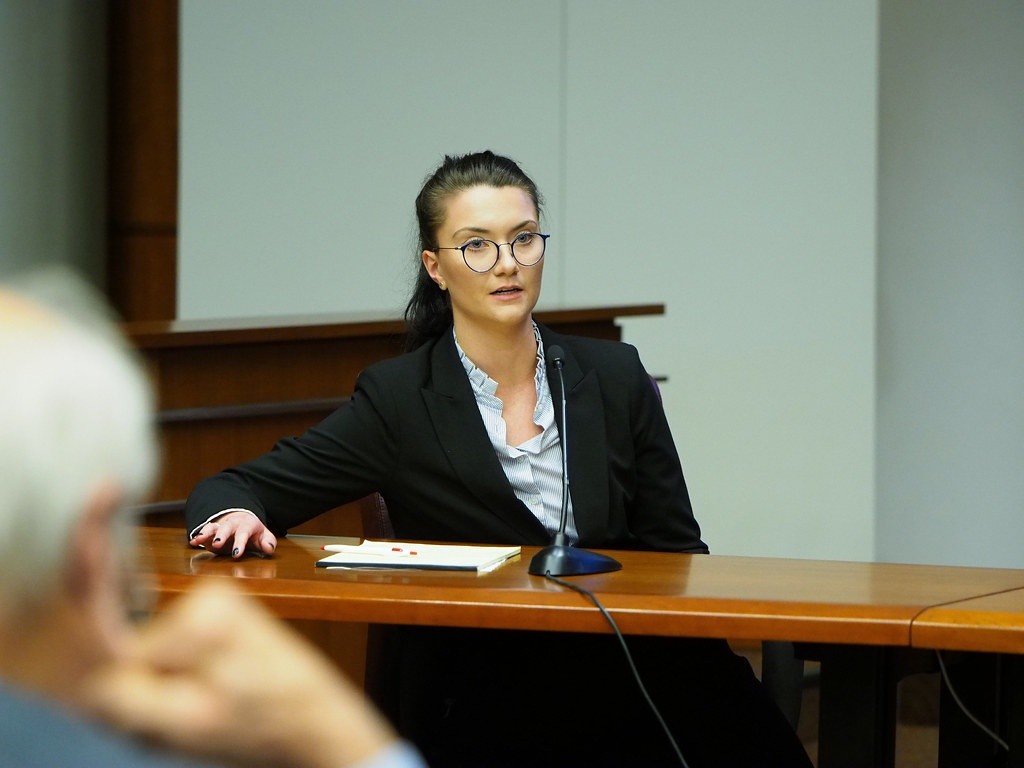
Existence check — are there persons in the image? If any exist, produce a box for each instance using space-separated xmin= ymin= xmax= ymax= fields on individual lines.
xmin=191 ymin=149 xmax=813 ymax=768
xmin=0 ymin=280 xmax=426 ymax=768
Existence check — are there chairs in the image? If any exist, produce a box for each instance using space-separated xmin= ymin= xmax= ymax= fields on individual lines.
xmin=360 ymin=490 xmax=804 ymax=736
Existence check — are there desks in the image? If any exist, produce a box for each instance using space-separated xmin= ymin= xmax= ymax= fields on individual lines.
xmin=129 ymin=520 xmax=1024 ymax=768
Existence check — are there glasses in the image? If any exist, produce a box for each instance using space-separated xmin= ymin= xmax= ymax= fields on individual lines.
xmin=431 ymin=232 xmax=550 ymax=273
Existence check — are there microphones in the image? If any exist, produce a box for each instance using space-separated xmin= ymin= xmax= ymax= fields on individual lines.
xmin=528 ymin=344 xmax=623 ymax=574
